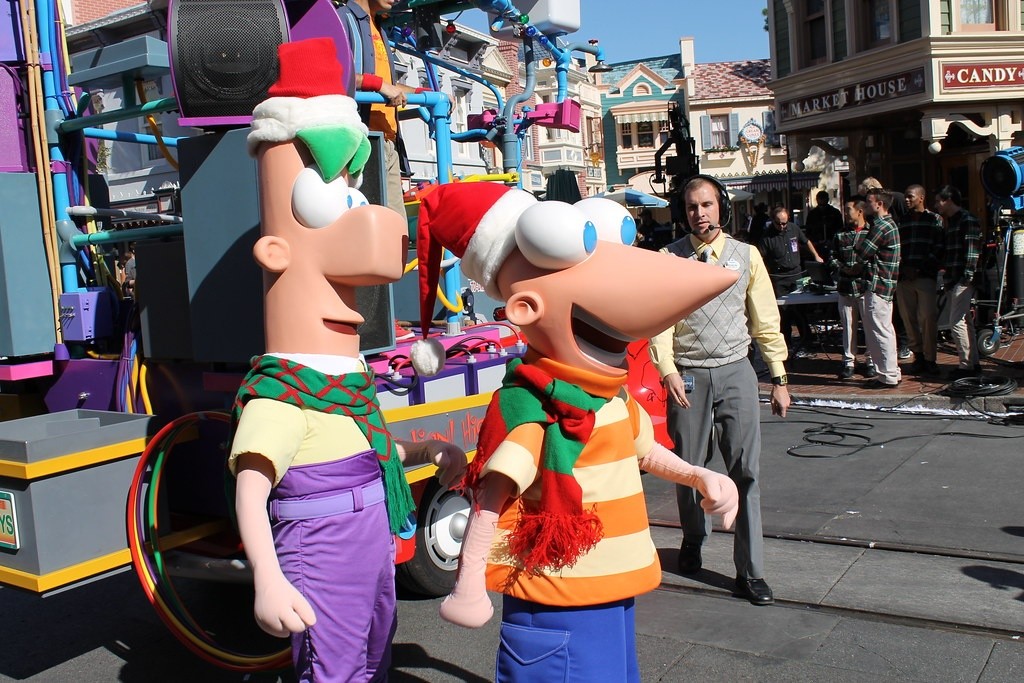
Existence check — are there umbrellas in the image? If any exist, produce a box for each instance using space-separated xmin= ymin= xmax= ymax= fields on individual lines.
xmin=584 ymin=186 xmax=669 ymax=208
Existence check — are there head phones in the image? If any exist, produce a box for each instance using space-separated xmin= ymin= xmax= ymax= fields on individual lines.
xmin=678 ymin=174 xmax=731 ymax=233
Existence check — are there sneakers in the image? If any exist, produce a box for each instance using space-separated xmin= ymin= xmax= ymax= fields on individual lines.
xmin=392 ymin=319 xmax=415 ymax=340
xmin=865 ymin=366 xmax=876 ymax=377
xmin=897 ymin=347 xmax=913 ymax=359
xmin=840 ymin=365 xmax=856 ymax=378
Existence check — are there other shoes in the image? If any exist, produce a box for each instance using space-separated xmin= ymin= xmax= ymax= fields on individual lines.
xmin=904 ymin=357 xmax=936 ymax=374
xmin=948 ymin=366 xmax=982 ymax=383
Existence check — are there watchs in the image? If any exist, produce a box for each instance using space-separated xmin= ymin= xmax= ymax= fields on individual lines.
xmin=771 ymin=375 xmax=787 ymax=385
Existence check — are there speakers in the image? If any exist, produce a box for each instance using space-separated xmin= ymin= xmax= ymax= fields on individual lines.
xmin=467 ymin=109 xmax=497 ymax=131
xmin=0 ymin=0 xmax=60 ymax=360
xmin=534 ymin=98 xmax=581 ymax=133
xmin=166 ymin=0 xmax=357 ymax=127
xmin=176 ymin=126 xmax=396 ymax=369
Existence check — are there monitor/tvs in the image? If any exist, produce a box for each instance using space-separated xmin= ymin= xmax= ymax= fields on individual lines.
xmin=806 ymin=259 xmax=835 ymax=296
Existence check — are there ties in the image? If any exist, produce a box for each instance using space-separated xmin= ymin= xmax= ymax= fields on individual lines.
xmin=703 ymin=244 xmax=714 ymax=264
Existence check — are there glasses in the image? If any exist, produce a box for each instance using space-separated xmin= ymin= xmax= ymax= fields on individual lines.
xmin=774 ymin=219 xmax=789 ymax=226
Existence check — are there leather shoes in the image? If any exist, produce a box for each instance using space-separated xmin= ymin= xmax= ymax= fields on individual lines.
xmin=862 ymin=377 xmax=902 ymax=389
xmin=735 ymin=571 xmax=775 ymax=605
xmin=677 ymin=536 xmax=702 ymax=573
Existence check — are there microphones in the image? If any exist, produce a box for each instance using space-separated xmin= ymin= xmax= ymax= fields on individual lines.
xmin=709 ymin=206 xmax=731 ymax=229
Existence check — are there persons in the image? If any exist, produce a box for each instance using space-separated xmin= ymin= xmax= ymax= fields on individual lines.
xmin=827 ymin=195 xmax=878 ymax=377
xmin=746 ymin=202 xmax=770 ymax=246
xmin=805 ymin=191 xmax=844 ymax=249
xmin=335 ymin=0 xmax=456 ymax=222
xmin=934 ymin=183 xmax=983 ymax=381
xmin=896 ymin=184 xmax=944 ymax=372
xmin=228 ymin=38 xmax=467 ymax=683
xmin=654 ymin=175 xmax=791 ymax=604
xmin=755 ymin=204 xmax=824 ymax=358
xmin=858 ymin=177 xmax=913 ymax=359
xmin=853 ymin=188 xmax=901 ymax=390
xmin=416 ymin=181 xmax=739 ymax=683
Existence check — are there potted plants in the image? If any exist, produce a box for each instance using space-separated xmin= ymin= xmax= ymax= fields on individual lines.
xmin=705 ymin=144 xmax=740 ymax=160
xmin=770 ymin=140 xmax=787 ymax=156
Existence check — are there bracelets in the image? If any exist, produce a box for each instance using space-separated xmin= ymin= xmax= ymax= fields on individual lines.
xmin=415 ymin=87 xmax=432 ymax=94
xmin=362 ymin=72 xmax=384 ymax=91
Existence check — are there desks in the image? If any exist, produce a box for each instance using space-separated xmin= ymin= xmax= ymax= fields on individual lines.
xmin=777 ymin=288 xmax=840 ymax=368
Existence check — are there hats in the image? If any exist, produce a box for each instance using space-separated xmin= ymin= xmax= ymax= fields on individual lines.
xmin=409 ymin=182 xmax=536 ymax=377
xmin=246 ymin=36 xmax=370 ymax=159
